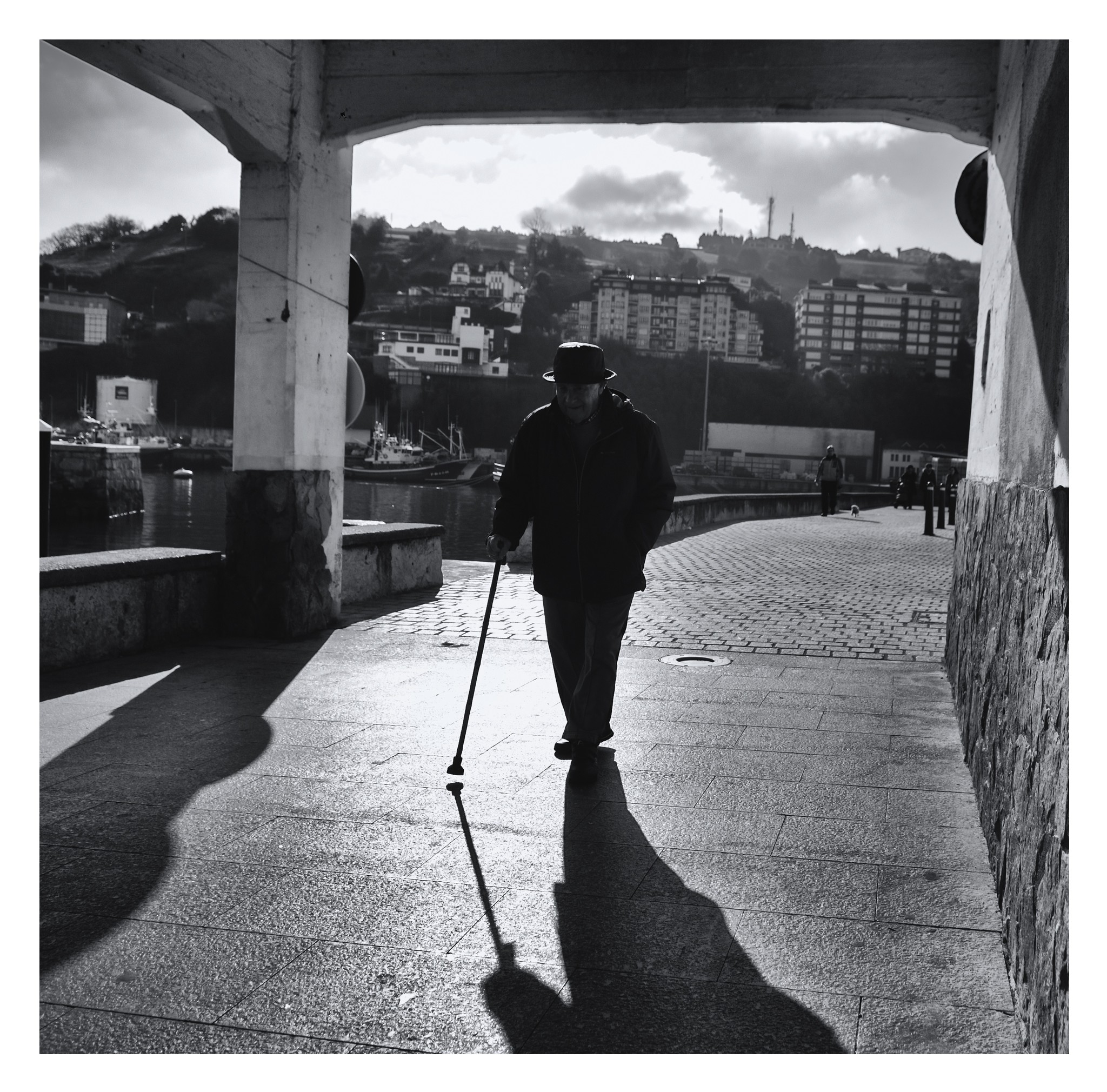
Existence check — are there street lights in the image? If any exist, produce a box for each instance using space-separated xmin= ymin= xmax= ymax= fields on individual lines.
xmin=701 ymin=337 xmax=717 ymax=452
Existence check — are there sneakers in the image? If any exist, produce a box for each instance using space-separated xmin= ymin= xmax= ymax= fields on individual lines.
xmin=553 ymin=728 xmax=613 ymax=753
xmin=567 ymin=741 xmax=598 ymax=785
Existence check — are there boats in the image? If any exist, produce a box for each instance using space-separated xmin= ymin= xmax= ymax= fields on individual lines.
xmin=173 ymin=468 xmax=193 ymax=477
xmin=50 ymin=370 xmax=182 ymax=468
xmin=672 ymin=450 xmax=891 ymax=495
xmin=344 ymin=397 xmax=494 ymax=487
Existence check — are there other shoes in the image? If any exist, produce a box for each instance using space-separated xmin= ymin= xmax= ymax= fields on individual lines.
xmin=831 ymin=508 xmax=835 ymax=515
xmin=821 ymin=512 xmax=827 ymax=516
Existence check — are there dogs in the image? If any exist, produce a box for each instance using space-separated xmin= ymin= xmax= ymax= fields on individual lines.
xmin=851 ymin=505 xmax=859 ymax=517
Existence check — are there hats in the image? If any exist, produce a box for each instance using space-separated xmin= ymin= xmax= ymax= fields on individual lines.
xmin=543 ymin=341 xmax=617 ymax=385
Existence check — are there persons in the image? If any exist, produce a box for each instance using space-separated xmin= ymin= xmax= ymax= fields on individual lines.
xmin=484 ymin=340 xmax=675 ymax=755
xmin=813 ymin=445 xmax=843 ymax=516
xmin=894 ymin=463 xmax=960 ymax=511
